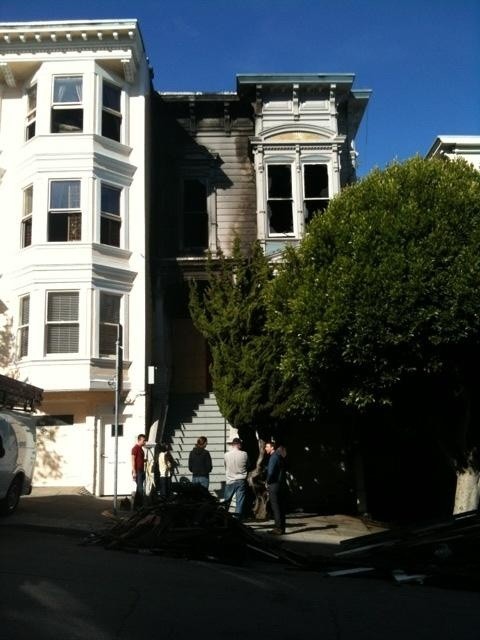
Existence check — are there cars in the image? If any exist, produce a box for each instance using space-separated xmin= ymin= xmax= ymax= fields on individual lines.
xmin=0 ymin=410 xmax=36 ymax=516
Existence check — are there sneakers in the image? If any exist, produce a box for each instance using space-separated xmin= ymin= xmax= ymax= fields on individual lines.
xmin=267 ymin=528 xmax=286 ymax=536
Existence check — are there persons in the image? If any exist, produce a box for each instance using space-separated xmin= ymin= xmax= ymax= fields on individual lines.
xmin=223 ymin=438 xmax=249 ymax=520
xmin=264 ymin=442 xmax=286 ymax=535
xmin=157 ymin=439 xmax=173 ymax=501
xmin=188 ymin=436 xmax=213 ymax=491
xmin=131 ymin=433 xmax=147 ymax=511
